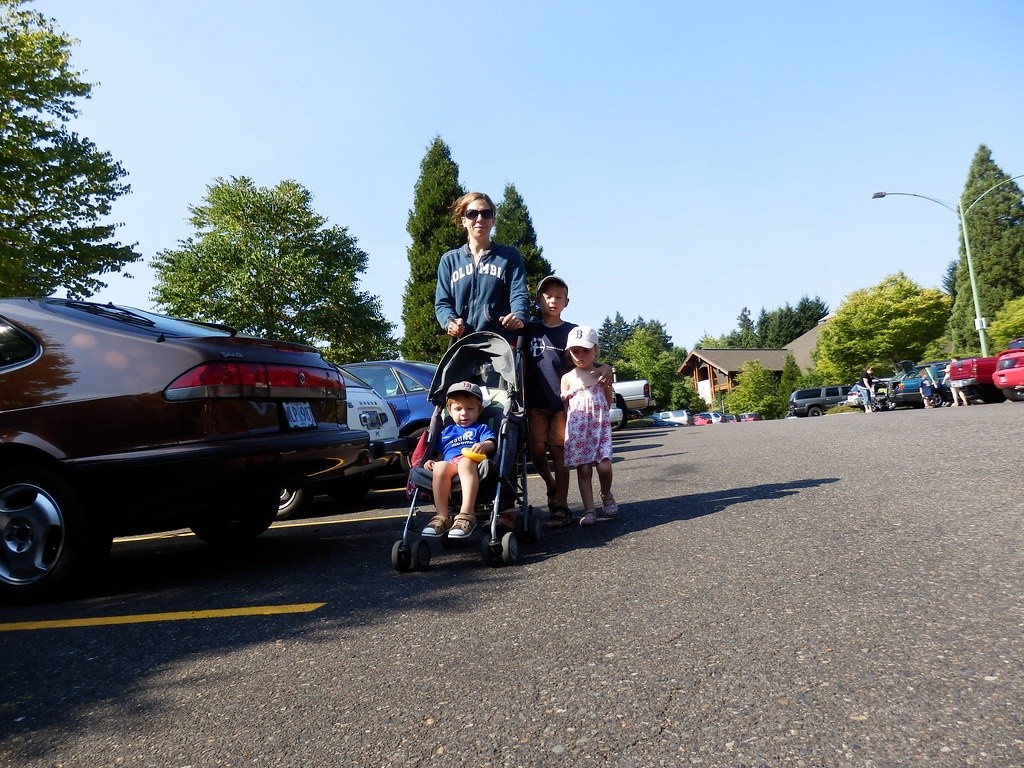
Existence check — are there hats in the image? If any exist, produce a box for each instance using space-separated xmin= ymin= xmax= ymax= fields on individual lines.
xmin=565 ymin=326 xmax=599 ymax=350
xmin=446 ymin=381 xmax=483 ymax=399
xmin=536 ymin=275 xmax=568 ymax=298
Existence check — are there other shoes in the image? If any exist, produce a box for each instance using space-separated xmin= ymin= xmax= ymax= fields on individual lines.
xmin=579 ymin=511 xmax=596 ymax=526
xmin=598 ymin=490 xmax=618 ymax=515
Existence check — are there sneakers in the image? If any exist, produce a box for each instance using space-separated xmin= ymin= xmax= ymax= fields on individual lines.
xmin=447 ymin=514 xmax=477 ymax=538
xmin=421 ymin=514 xmax=453 ymax=537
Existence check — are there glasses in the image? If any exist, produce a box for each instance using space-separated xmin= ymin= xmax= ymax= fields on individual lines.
xmin=463 ymin=208 xmax=495 ymax=219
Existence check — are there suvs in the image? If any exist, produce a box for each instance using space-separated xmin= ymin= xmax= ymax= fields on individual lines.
xmin=787 ymin=385 xmax=853 ymax=418
xmin=991 ymin=337 xmax=1024 ymax=402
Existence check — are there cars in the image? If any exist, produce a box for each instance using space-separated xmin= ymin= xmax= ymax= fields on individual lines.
xmin=849 ymin=360 xmax=987 ymax=412
xmin=273 ymin=361 xmax=418 ymax=523
xmin=0 ymin=291 xmax=372 ymax=611
xmin=652 ymin=408 xmax=761 ymax=427
xmin=847 ymin=380 xmax=865 ymax=411
xmin=334 ymin=360 xmax=438 ymax=478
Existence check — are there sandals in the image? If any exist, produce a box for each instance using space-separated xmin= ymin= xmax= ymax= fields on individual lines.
xmin=546 ymin=487 xmax=557 ymax=514
xmin=544 ymin=505 xmax=574 ymax=527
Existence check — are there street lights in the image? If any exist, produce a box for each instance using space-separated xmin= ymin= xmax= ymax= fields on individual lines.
xmin=871 ymin=174 xmax=1024 ymax=358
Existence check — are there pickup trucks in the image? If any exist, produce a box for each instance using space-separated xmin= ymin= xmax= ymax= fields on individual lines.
xmin=949 ymin=356 xmax=1008 ymax=404
xmin=611 ymin=379 xmax=656 ymax=433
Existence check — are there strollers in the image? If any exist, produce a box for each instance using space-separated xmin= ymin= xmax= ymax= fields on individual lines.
xmin=389 ymin=315 xmax=540 ymax=575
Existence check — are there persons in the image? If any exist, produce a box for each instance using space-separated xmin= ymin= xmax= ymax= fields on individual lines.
xmin=918 ymin=372 xmax=935 ymax=409
xmin=524 ymin=275 xmax=614 ymax=528
xmin=943 ymin=357 xmax=968 ymax=406
xmin=435 ymin=192 xmax=531 ymax=374
xmin=560 ymin=326 xmax=618 ymax=525
xmin=856 ymin=367 xmax=879 ymax=413
xmin=421 ymin=381 xmax=497 ymax=540
